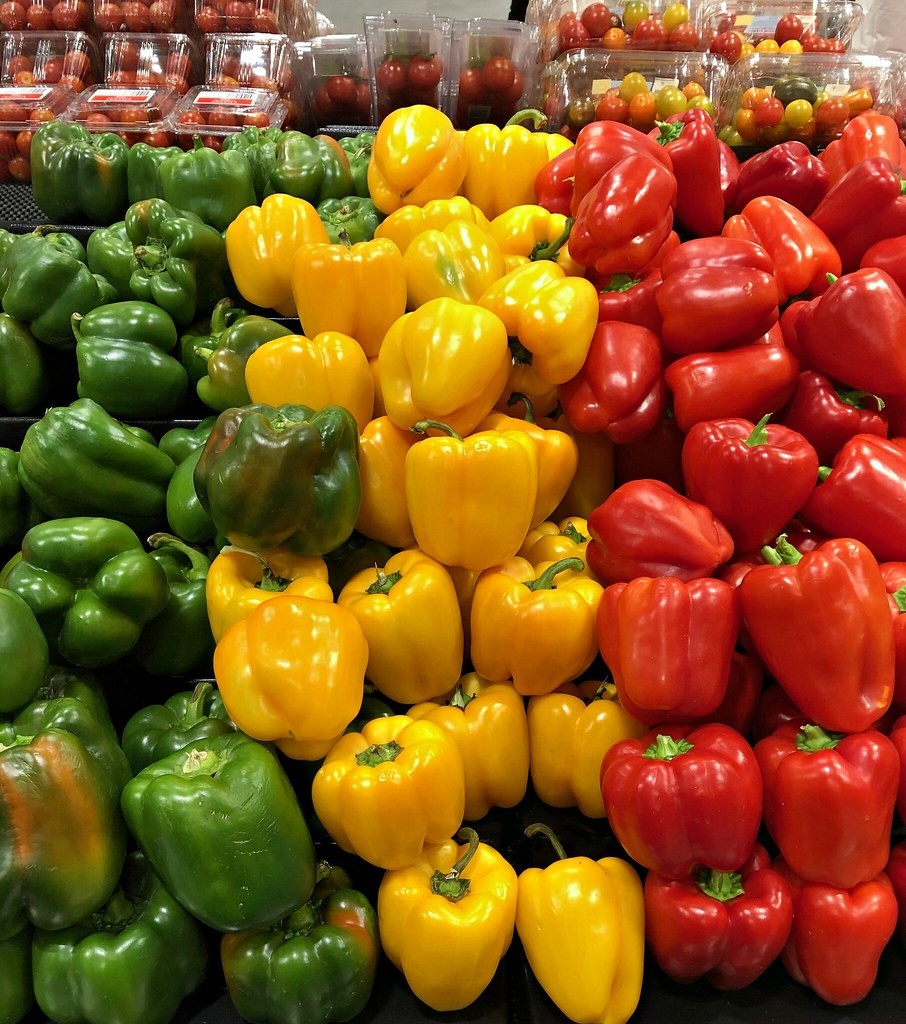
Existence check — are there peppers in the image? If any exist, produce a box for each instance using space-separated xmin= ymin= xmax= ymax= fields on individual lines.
xmin=0 ymin=102 xmax=906 ymax=1024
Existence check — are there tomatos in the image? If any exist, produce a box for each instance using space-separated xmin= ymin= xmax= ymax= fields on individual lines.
xmin=0 ymin=0 xmax=906 ymax=184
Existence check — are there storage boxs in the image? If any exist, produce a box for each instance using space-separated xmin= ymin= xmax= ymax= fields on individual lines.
xmin=101 ymin=33 xmax=203 ymax=92
xmin=294 ymin=32 xmax=373 ymax=126
xmin=721 ymin=51 xmax=906 ymax=140
xmin=200 ymin=33 xmax=316 ymax=135
xmin=550 ymin=47 xmax=727 ymax=134
xmin=1 ymin=31 xmax=103 ymax=93
xmin=0 ymin=84 xmax=75 ymax=182
xmin=698 ymin=0 xmax=863 ymax=53
xmin=362 ymin=10 xmax=452 ymax=124
xmin=171 ymin=86 xmax=290 ymax=150
xmin=0 ymin=0 xmax=100 ymax=36
xmin=193 ymin=0 xmax=341 ymax=43
xmin=91 ymin=0 xmax=193 ymax=34
xmin=64 ymin=83 xmax=178 ymax=148
xmin=447 ymin=16 xmax=537 ymax=130
xmin=525 ymin=0 xmax=714 ymax=61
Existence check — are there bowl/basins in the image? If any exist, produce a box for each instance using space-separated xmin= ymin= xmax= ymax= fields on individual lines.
xmin=0 ymin=0 xmax=906 ymax=154
